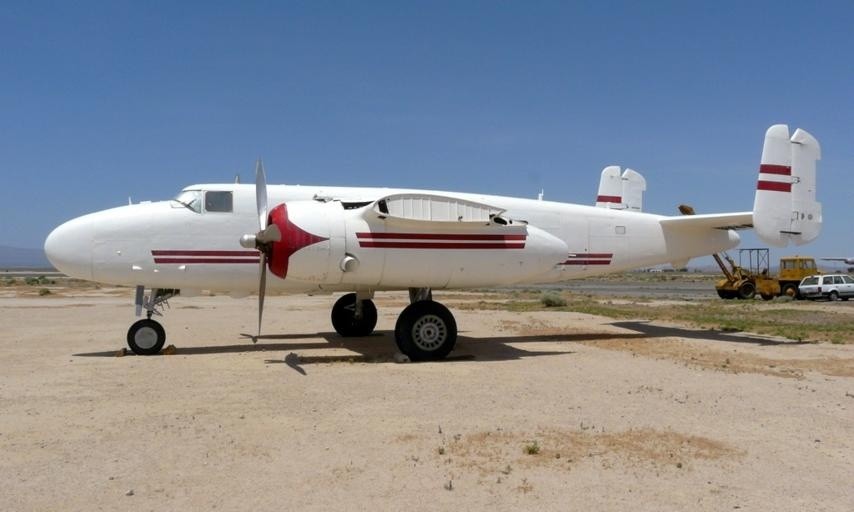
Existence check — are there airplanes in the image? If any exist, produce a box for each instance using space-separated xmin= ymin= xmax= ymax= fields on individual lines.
xmin=43 ymin=119 xmax=823 ymax=361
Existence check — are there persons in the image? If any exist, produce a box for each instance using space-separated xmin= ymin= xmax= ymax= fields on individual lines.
xmin=759 ymin=268 xmax=769 ymax=279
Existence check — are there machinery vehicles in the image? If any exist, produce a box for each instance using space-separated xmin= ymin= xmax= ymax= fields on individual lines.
xmin=678 ymin=202 xmax=821 ymax=300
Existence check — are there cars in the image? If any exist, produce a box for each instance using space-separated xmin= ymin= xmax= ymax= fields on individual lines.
xmin=797 ymin=274 xmax=854 ymax=301
